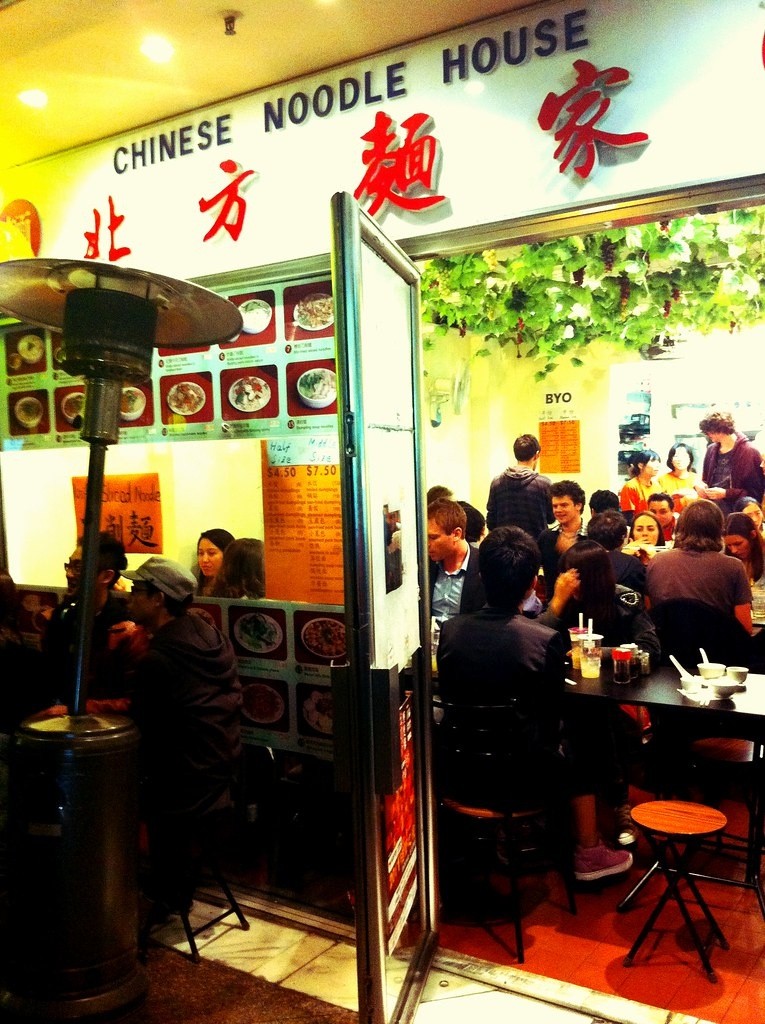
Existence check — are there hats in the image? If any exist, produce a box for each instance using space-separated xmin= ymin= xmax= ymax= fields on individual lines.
xmin=119 ymin=556 xmax=196 ymax=601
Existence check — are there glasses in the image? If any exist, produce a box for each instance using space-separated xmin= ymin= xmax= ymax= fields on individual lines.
xmin=131 ymin=585 xmax=152 ymax=596
xmin=64 ymin=563 xmax=84 ymax=575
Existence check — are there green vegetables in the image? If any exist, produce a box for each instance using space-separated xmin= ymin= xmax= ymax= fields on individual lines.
xmin=299 ymin=371 xmax=323 ymax=398
xmin=239 ymin=612 xmax=276 ymax=649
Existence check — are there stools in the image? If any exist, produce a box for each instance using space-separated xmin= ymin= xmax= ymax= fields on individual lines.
xmin=136 ymin=782 xmax=247 ymax=964
xmin=444 ymin=739 xmax=765 ymax=975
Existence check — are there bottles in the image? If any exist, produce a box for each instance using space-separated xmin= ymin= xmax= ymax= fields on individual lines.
xmin=611 ymin=643 xmax=650 ymax=683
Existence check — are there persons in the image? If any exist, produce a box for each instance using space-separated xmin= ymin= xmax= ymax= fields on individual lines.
xmin=212 ymin=538 xmax=265 ymax=599
xmin=383 ymin=411 xmax=765 ymax=749
xmin=437 ymin=528 xmax=632 ymax=882
xmin=196 ymin=529 xmax=236 ymax=596
xmin=0 ymin=531 xmax=240 ymax=925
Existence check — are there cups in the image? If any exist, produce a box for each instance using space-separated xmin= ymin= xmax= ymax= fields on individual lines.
xmin=727 ymin=667 xmax=749 ymax=684
xmin=578 ymin=633 xmax=605 ymax=678
xmin=568 ymin=627 xmax=594 ymax=670
xmin=681 ymin=675 xmax=701 ymax=694
xmin=751 ymin=590 xmax=765 ymax=617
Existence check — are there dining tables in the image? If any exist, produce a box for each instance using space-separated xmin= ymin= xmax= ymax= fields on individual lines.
xmin=433 ymin=641 xmax=765 ymax=922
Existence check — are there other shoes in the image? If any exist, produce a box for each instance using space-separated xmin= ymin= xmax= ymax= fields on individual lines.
xmin=137 ymin=888 xmax=196 ymax=915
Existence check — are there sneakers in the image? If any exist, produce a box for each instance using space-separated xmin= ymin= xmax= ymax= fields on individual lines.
xmin=611 ymin=802 xmax=640 ymax=845
xmin=574 ymin=839 xmax=633 ymax=880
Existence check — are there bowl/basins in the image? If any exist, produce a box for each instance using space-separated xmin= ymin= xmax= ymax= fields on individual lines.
xmin=709 ymin=679 xmax=740 ymax=698
xmin=698 ymin=663 xmax=726 ymax=682
xmin=297 ymin=368 xmax=338 ymax=409
xmin=120 ymin=387 xmax=147 ymax=421
xmin=8 ymin=334 xmax=87 ymax=429
xmin=238 ymin=299 xmax=273 ymax=333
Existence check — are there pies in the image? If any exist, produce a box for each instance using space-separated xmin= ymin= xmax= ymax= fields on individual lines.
xmin=297 ymin=295 xmax=333 ymax=329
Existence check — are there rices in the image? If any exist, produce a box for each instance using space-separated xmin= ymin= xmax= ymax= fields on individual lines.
xmin=18 ymin=336 xmax=44 ymax=358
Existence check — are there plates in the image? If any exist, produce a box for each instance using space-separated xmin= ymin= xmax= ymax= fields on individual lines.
xmin=237 ymin=684 xmax=285 ymax=723
xmin=292 ymin=293 xmax=335 ymax=331
xmin=301 ymin=618 xmax=348 ymax=658
xmin=303 ymin=692 xmax=333 ymax=736
xmin=228 ymin=377 xmax=271 ymax=413
xmin=167 ymin=381 xmax=205 ymax=417
xmin=234 ymin=614 xmax=283 ymax=653
